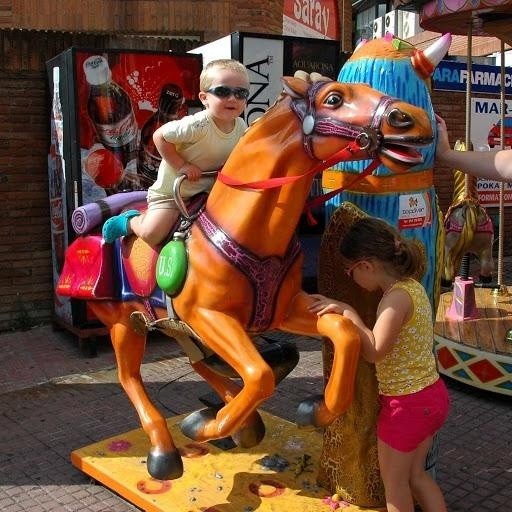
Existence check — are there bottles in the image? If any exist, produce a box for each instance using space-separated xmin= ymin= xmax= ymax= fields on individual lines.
xmin=49 ymin=144 xmax=66 ymax=278
xmin=81 ymin=55 xmax=137 ymax=197
xmin=50 ymin=67 xmax=64 ymax=156
xmin=138 ymin=83 xmax=185 ymax=191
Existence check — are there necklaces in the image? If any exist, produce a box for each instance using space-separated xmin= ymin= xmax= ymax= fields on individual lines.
xmin=383 ymin=279 xmax=401 ymax=297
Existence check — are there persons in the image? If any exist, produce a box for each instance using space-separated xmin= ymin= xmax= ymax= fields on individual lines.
xmin=101 ymin=58 xmax=255 ymax=247
xmin=431 ymin=111 xmax=512 ymax=193
xmin=306 ymin=215 xmax=447 ymax=512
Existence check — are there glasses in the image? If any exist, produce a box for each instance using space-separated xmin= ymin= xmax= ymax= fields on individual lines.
xmin=205 ymin=86 xmax=249 ymax=100
xmin=343 ymin=256 xmax=378 ymax=277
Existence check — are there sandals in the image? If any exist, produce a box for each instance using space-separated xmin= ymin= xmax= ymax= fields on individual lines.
xmin=102 ymin=210 xmax=142 ymax=243
